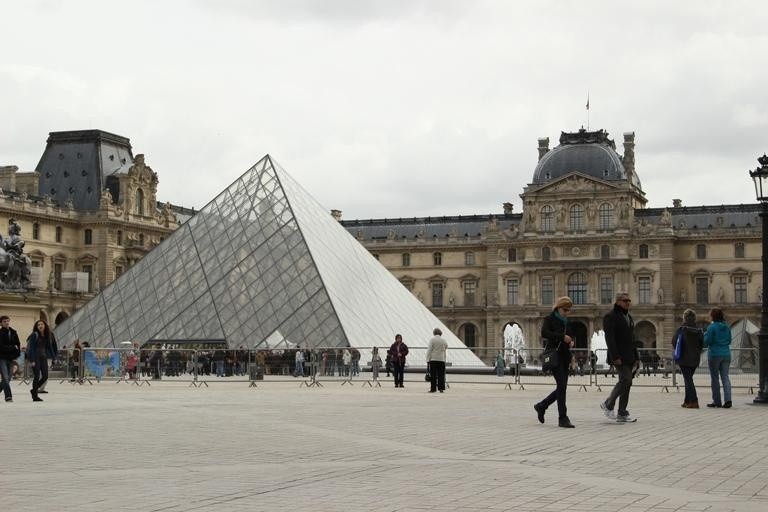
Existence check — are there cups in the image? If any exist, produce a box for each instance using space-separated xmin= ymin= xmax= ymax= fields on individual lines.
xmin=620 ymin=298 xmax=631 ymax=303
xmin=562 ymin=308 xmax=570 ymax=312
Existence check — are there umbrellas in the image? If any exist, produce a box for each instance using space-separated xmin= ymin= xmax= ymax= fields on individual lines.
xmin=559 ymin=416 xmax=575 ymax=427
xmin=722 ymin=401 xmax=732 ymax=407
xmin=708 ymin=402 xmax=721 ymax=407
xmin=534 ymin=403 xmax=545 ymax=423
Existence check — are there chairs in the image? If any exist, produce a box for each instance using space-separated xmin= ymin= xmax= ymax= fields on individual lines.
xmin=31 ymin=389 xmax=33 ymax=394
xmin=682 ymin=403 xmax=698 ymax=407
xmin=617 ymin=414 xmax=637 ymax=422
xmin=38 ymin=390 xmax=48 ymax=393
xmin=600 ymin=402 xmax=617 ymax=421
xmin=6 ymin=397 xmax=12 ymax=402
xmin=32 ymin=396 xmax=43 ymax=401
xmin=400 ymin=384 xmax=404 ymax=387
xmin=395 ymin=384 xmax=398 ymax=387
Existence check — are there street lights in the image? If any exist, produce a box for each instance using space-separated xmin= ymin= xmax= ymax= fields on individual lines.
xmin=518 ymin=354 xmax=524 ymax=364
xmin=607 ymin=344 xmax=632 ymax=364
xmin=542 ymin=349 xmax=560 ymax=372
xmin=674 ymin=327 xmax=681 ymax=360
xmin=425 ymin=374 xmax=432 ymax=381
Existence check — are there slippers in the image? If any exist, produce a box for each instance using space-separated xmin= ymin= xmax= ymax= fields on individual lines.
xmin=25 ymin=332 xmax=38 ymax=363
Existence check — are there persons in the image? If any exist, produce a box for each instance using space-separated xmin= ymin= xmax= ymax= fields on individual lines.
xmin=671 ymin=308 xmax=704 ymax=409
xmin=640 ymin=350 xmax=650 ymax=376
xmin=49 ymin=344 xmax=70 ymax=371
xmin=702 ymin=307 xmax=732 ymax=409
xmin=80 ymin=341 xmax=100 ymax=383
xmin=388 ymin=334 xmax=409 ymax=388
xmin=385 ymin=350 xmax=395 ymax=377
xmin=69 ymin=339 xmax=82 ymax=381
xmin=140 ymin=342 xmax=361 ymax=380
xmin=605 ymin=364 xmax=615 ymax=378
xmin=534 ymin=296 xmax=576 ymax=428
xmin=0 ymin=316 xmax=20 ymax=402
xmin=510 ymin=349 xmax=519 ymax=378
xmin=599 ymin=292 xmax=641 ymax=423
xmin=650 ymin=350 xmax=661 ymax=377
xmin=370 ymin=347 xmax=383 ymax=377
xmin=577 ymin=351 xmax=587 ymax=376
xmin=568 ymin=351 xmax=576 ymax=376
xmin=539 ymin=349 xmax=552 ymax=376
xmin=426 ymin=328 xmax=448 ymax=393
xmin=495 ymin=349 xmax=506 ymax=376
xmin=24 ymin=319 xmax=58 ymax=403
xmin=126 ymin=341 xmax=139 ymax=379
xmin=589 ymin=350 xmax=598 ymax=374
xmin=2 ymin=216 xmax=26 ymax=279
xmin=36 ymin=325 xmax=56 ymax=393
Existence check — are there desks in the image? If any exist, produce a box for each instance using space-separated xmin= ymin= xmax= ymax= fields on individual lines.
xmin=748 ymin=151 xmax=767 ymax=407
